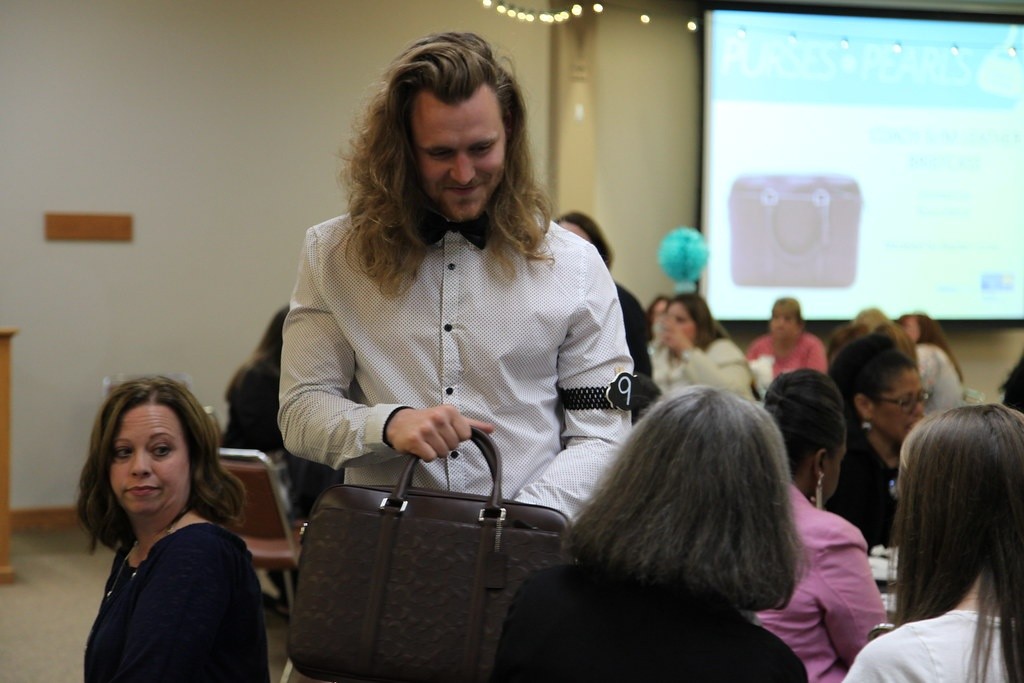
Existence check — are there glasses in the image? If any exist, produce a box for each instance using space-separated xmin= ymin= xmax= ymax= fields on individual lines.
xmin=878 ymin=389 xmax=929 ymax=408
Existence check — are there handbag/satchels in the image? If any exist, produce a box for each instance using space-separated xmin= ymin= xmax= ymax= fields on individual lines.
xmin=286 ymin=426 xmax=570 ymax=683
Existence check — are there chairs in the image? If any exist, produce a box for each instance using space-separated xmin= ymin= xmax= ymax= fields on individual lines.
xmin=217 ymin=447 xmax=308 ymax=683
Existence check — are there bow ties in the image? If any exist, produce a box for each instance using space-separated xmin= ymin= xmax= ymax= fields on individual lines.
xmin=418 ymin=210 xmax=489 ymax=250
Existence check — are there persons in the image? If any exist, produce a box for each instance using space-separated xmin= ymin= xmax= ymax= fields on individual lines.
xmin=76 ymin=374 xmax=271 ymax=683
xmin=221 ymin=213 xmax=1024 ymax=682
xmin=277 ymin=30 xmax=634 ymax=528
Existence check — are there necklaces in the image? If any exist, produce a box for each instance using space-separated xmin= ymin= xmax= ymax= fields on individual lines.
xmin=105 ymin=521 xmax=177 ymax=604
